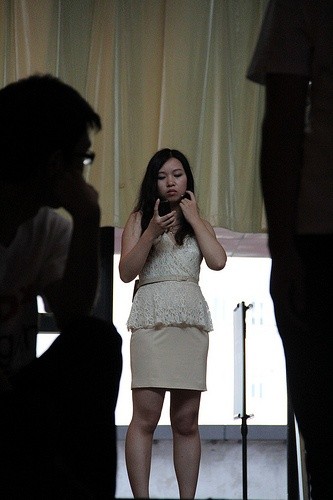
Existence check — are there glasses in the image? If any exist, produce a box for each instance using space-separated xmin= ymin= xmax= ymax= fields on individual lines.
xmin=58 ymin=149 xmax=95 ymax=165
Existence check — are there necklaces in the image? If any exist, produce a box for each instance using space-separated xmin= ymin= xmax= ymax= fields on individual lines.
xmin=169 ymin=227 xmax=174 ymax=235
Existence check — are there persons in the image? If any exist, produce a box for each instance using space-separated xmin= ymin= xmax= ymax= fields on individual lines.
xmin=119 ymin=149 xmax=227 ymax=500
xmin=246 ymin=0 xmax=333 ymax=500
xmin=0 ymin=76 xmax=122 ymax=500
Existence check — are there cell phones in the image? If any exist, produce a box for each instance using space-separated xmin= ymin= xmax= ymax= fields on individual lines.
xmin=158 ymin=199 xmax=171 ymax=217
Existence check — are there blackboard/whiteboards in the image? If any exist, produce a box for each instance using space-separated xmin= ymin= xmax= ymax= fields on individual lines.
xmin=233 ymin=301 xmax=248 ymax=420
